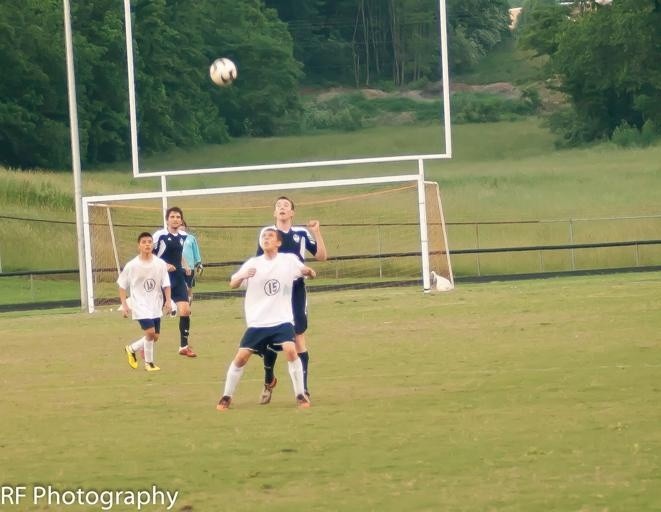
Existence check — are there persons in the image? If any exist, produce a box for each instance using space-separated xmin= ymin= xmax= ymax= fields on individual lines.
xmin=141 ymin=208 xmax=197 ymax=358
xmin=255 ymin=197 xmax=327 ymax=405
xmin=117 ymin=232 xmax=173 ymax=370
xmin=179 ymin=217 xmax=204 ymax=313
xmin=218 ymin=227 xmax=317 ymax=411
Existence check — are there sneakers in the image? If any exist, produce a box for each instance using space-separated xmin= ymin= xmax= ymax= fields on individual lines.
xmin=216 ymin=395 xmax=231 ymax=411
xmin=295 ymin=392 xmax=311 ymax=409
xmin=178 ymin=345 xmax=196 ymax=358
xmin=143 ymin=362 xmax=160 ymax=372
xmin=125 ymin=345 xmax=138 ymax=368
xmin=260 ymin=376 xmax=277 ymax=404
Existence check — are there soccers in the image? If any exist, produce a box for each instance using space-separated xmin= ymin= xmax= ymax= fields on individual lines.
xmin=209 ymin=57 xmax=238 ymax=86
